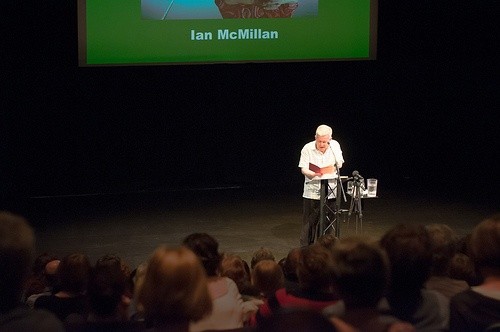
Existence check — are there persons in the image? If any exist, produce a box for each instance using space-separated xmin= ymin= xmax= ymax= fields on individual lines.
xmin=298 ymin=124 xmax=344 ymax=248
xmin=0 ymin=208 xmax=499 ymax=332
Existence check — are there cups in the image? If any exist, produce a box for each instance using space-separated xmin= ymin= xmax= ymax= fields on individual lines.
xmin=367 ymin=178 xmax=377 ymax=198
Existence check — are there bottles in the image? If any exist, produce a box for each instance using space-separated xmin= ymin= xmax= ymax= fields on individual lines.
xmin=347 ymin=177 xmax=355 ymax=194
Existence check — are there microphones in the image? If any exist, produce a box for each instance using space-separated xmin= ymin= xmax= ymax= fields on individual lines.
xmin=328 ymin=142 xmax=338 ymax=165
xmin=352 ymin=171 xmax=363 ymax=179
